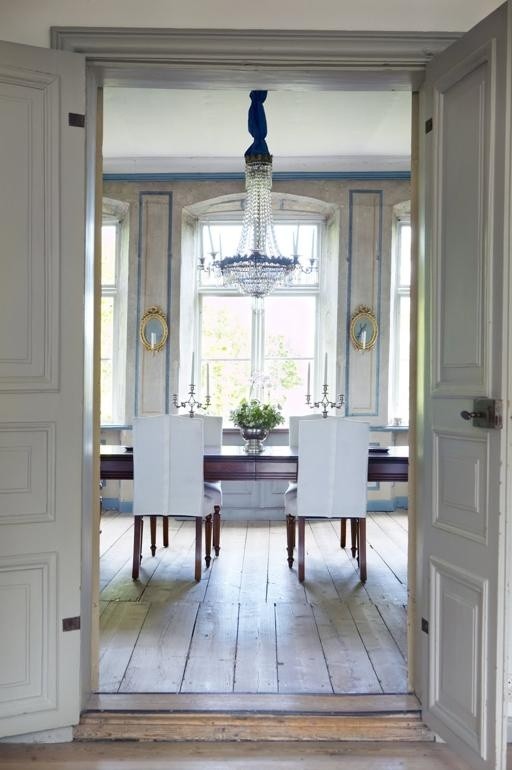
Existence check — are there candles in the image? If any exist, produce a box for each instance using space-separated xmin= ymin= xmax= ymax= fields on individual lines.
xmin=306 ymin=352 xmax=346 ymax=397
xmin=172 ymin=351 xmax=210 ymax=398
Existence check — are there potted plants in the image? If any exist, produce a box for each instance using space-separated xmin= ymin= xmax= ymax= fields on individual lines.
xmin=231 ymin=395 xmax=284 ymax=449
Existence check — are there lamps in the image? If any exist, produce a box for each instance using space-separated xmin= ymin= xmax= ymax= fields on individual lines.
xmin=193 ymin=87 xmax=323 ymax=301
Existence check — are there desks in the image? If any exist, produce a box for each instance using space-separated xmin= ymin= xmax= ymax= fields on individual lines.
xmin=98 ymin=443 xmax=410 ymax=486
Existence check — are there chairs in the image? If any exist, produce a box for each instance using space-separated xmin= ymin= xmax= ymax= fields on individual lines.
xmin=284 ymin=411 xmax=371 ymax=584
xmin=129 ymin=412 xmax=224 ymax=584
